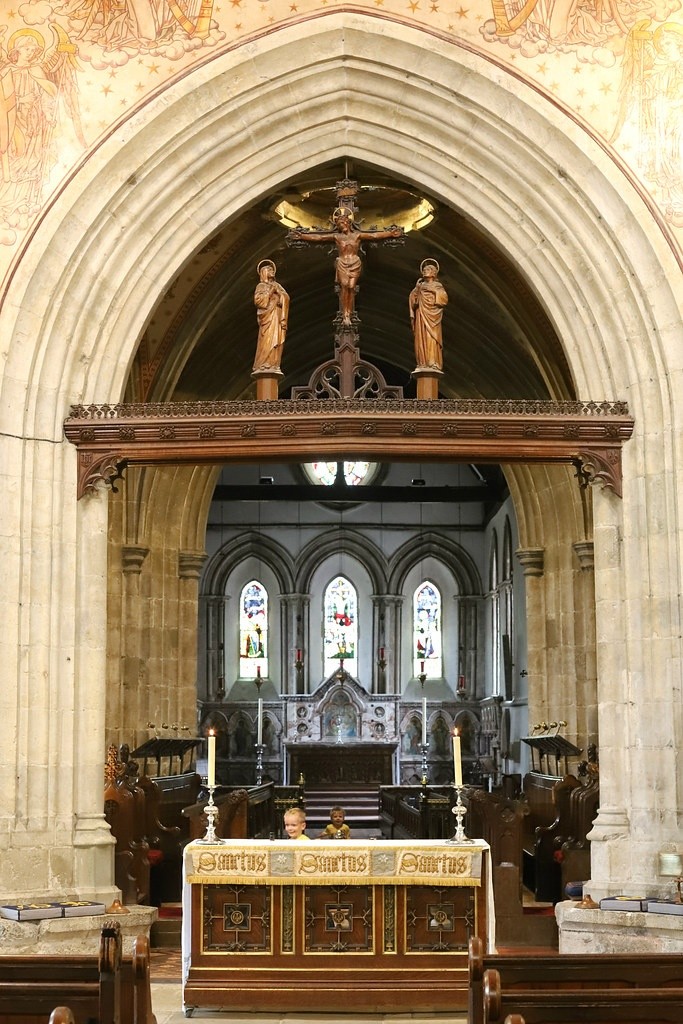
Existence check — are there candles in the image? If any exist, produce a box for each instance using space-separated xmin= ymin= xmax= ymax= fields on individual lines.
xmin=421 ymin=698 xmax=426 ymax=745
xmin=453 ymin=728 xmax=463 ymax=786
xmin=219 ymin=678 xmax=222 ymax=689
xmin=460 ymin=677 xmax=464 ymax=686
xmin=298 ymin=649 xmax=300 ymax=661
xmin=258 ymin=666 xmax=260 ymax=674
xmin=421 ymin=662 xmax=424 ymax=672
xmin=381 ymin=648 xmax=384 ymax=658
xmin=258 ymin=698 xmax=263 ymax=746
xmin=208 ymin=728 xmax=216 ymax=786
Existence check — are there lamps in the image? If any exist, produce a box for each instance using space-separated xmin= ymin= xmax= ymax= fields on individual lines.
xmin=260 ymin=477 xmax=274 ymax=484
xmin=412 ymin=478 xmax=426 ymax=486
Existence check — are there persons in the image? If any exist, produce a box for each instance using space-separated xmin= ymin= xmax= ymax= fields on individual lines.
xmin=408 ymin=265 xmax=448 ymax=372
xmin=292 ymin=215 xmax=401 ymax=324
xmin=284 ymin=808 xmax=311 ymax=840
xmin=252 ymin=266 xmax=290 ymax=374
xmin=321 ymin=807 xmax=352 ymax=839
